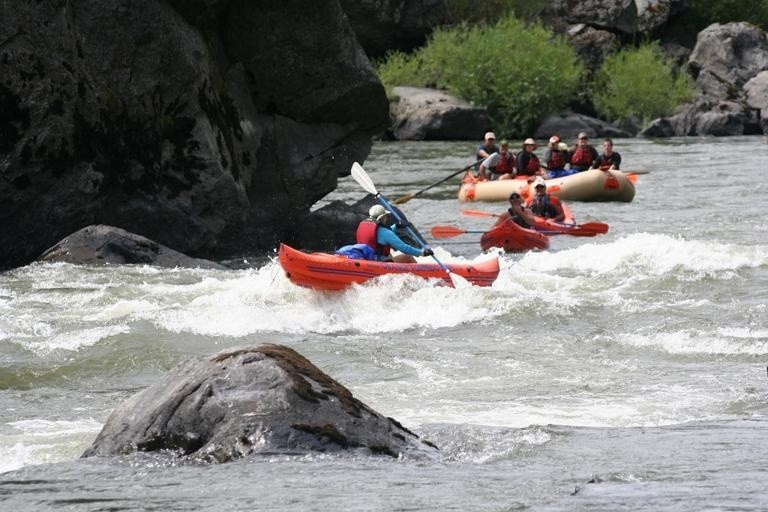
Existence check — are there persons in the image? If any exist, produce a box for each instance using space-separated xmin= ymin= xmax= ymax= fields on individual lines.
xmin=516 ymin=137 xmax=546 ymax=177
xmin=566 ymin=132 xmax=599 ymax=171
xmin=541 ymin=135 xmax=573 ymax=171
xmin=528 ymin=178 xmax=566 ymax=223
xmin=479 ymin=139 xmax=517 ymax=181
xmin=592 ymin=137 xmax=621 ymax=171
xmin=495 ymin=189 xmax=536 ymax=230
xmin=354 ymin=204 xmax=434 ymax=261
xmin=476 ymin=131 xmax=500 ymax=170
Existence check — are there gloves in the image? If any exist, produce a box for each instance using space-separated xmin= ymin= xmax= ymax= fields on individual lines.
xmin=423 ymin=249 xmax=433 ymax=256
xmin=395 ymin=220 xmax=411 ymax=228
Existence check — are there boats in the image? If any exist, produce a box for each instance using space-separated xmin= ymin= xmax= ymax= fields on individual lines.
xmin=525 ymin=203 xmax=609 ymax=236
xmin=279 ymin=242 xmax=499 ymax=292
xmin=478 ymin=220 xmax=549 ymax=251
xmin=458 ymin=170 xmax=635 ymax=202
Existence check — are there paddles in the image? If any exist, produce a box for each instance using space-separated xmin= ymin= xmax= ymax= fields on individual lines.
xmin=390 ymin=157 xmax=486 ymax=205
xmin=350 ymin=160 xmax=451 ymax=277
xmin=430 ymin=225 xmax=599 ymax=239
xmin=458 ymin=207 xmax=609 ymax=235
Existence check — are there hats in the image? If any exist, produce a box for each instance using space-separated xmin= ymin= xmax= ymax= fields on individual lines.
xmin=508 ymin=190 xmax=520 ymax=198
xmin=484 ymin=132 xmax=587 ymax=146
xmin=533 ymin=179 xmax=544 ymax=187
xmin=368 ymin=204 xmax=390 ymax=220
